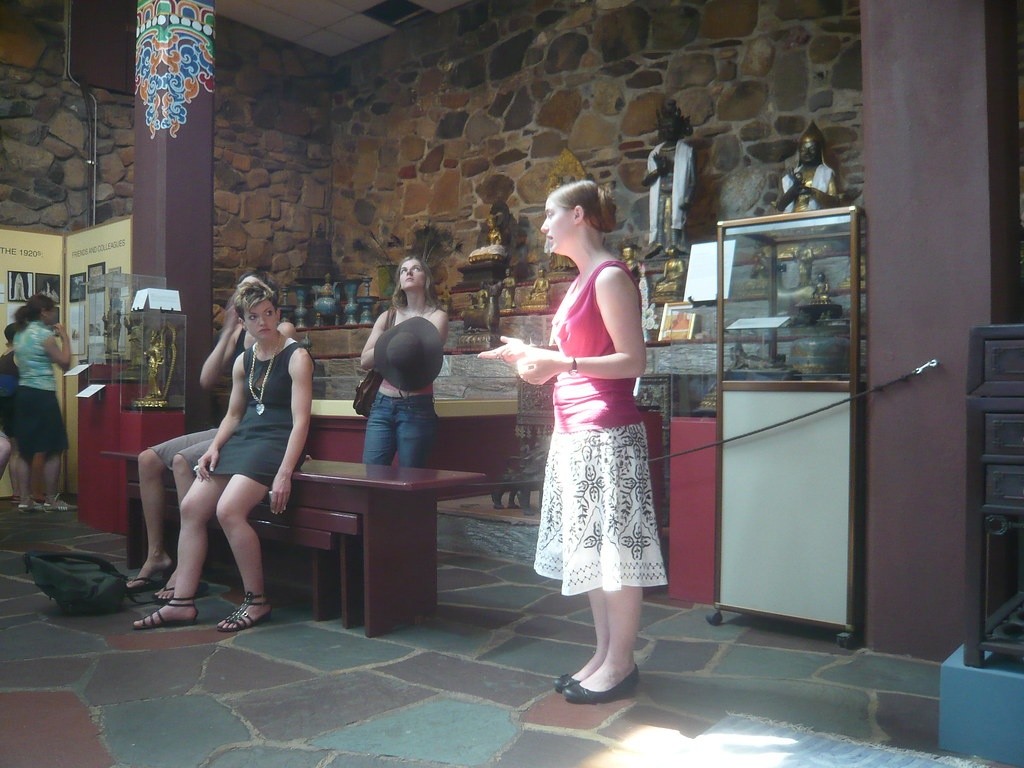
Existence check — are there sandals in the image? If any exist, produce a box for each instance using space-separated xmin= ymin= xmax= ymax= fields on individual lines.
xmin=217 ymin=592 xmax=273 ymax=632
xmin=132 ymin=597 xmax=199 ymax=631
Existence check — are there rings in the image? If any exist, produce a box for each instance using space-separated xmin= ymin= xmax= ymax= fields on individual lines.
xmin=495 ymin=354 xmax=501 ymax=360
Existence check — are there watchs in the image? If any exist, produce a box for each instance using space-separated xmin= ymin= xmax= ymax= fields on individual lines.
xmin=568 ymin=356 xmax=579 ymax=377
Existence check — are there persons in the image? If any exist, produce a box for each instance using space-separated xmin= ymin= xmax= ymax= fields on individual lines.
xmin=474 ymin=180 xmax=669 ymax=705
xmin=0 ymin=293 xmax=79 ymax=513
xmin=100 ymin=296 xmax=164 ymax=400
xmin=123 ymin=272 xmax=296 ymax=607
xmin=130 ymin=281 xmax=316 ymax=632
xmin=359 ymin=255 xmax=448 ymax=466
xmin=466 ymin=100 xmax=866 ymax=311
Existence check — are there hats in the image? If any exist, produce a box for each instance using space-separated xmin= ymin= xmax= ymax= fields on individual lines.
xmin=374 ymin=316 xmax=444 ymax=392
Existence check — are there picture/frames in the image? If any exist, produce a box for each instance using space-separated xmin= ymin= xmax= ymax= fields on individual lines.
xmin=87 ymin=262 xmax=105 ymax=294
xmin=7 ymin=270 xmax=34 ymax=301
xmin=108 ymin=266 xmax=121 ymax=298
xmin=70 ymin=272 xmax=86 ymax=302
xmin=35 ymin=273 xmax=60 ymax=301
xmin=658 ymin=301 xmax=695 ymax=338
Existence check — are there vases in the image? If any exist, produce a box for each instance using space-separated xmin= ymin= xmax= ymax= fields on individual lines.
xmin=281 ymin=305 xmax=296 ymax=324
xmin=356 ymin=296 xmax=378 ymax=325
xmin=342 ymin=278 xmax=361 ymax=324
xmin=293 ymin=284 xmax=308 ymax=328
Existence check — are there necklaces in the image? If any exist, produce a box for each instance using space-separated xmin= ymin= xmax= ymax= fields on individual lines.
xmin=249 ymin=331 xmax=283 ymax=416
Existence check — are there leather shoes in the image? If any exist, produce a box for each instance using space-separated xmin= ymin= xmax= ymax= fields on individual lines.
xmin=562 ymin=662 xmax=639 ymax=704
xmin=554 ymin=672 xmax=583 ymax=694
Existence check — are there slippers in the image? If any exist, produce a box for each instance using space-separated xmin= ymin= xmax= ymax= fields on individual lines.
xmin=151 ymin=581 xmax=209 ymax=604
xmin=124 ymin=578 xmax=166 ymax=595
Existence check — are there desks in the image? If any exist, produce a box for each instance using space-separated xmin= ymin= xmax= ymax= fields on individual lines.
xmin=100 ymin=448 xmax=486 ymax=639
xmin=964 ymin=324 xmax=1024 ymax=670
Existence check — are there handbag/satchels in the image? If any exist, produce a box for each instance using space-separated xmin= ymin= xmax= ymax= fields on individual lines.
xmin=352 ymin=369 xmax=380 ymax=419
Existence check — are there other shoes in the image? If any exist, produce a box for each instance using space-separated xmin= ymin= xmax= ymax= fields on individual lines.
xmin=10 ymin=496 xmax=22 ymax=504
xmin=42 ymin=496 xmax=78 ymax=513
xmin=18 ymin=498 xmax=44 ymax=512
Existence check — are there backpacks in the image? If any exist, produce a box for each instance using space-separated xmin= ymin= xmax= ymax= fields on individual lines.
xmin=20 ymin=550 xmax=128 ymax=615
